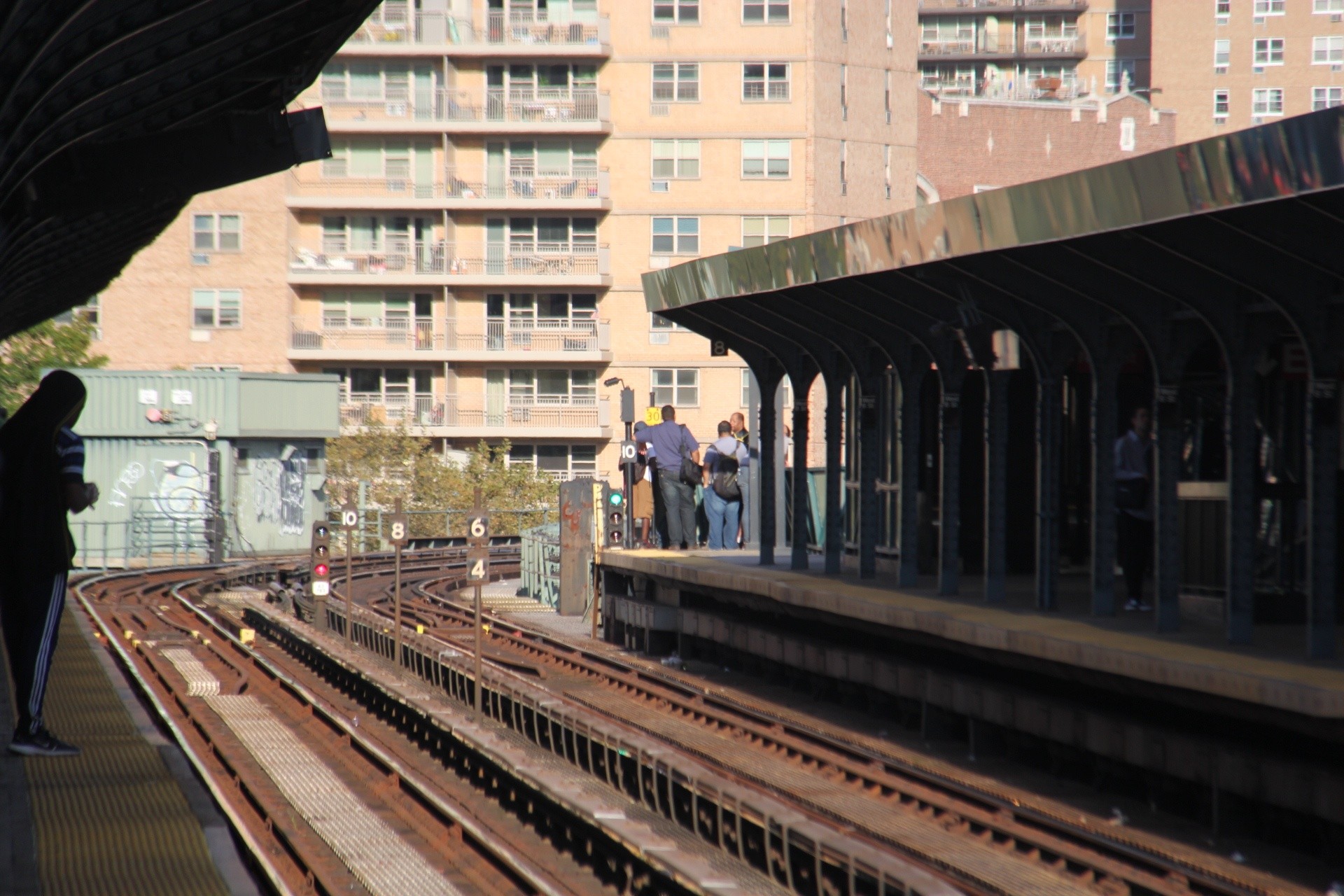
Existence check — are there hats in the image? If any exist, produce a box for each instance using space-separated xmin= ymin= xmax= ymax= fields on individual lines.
xmin=634 ymin=421 xmax=649 ymax=430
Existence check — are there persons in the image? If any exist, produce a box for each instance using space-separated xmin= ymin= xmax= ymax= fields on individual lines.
xmin=630 ymin=403 xmax=753 ymax=555
xmin=0 ymin=369 xmax=99 ymax=757
xmin=1113 ymin=406 xmax=1153 ymax=610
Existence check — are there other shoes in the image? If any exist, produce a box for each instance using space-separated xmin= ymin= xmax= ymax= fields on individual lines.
xmin=669 ymin=545 xmax=680 ymax=550
xmin=7 ymin=729 xmax=80 ymax=757
xmin=688 ymin=545 xmax=701 ymax=551
xmin=639 ymin=539 xmax=654 ymax=549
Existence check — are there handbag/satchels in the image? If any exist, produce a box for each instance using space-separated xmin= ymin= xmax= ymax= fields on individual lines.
xmin=679 ymin=458 xmax=702 ymax=486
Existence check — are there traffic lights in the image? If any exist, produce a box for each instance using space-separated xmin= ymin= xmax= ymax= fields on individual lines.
xmin=312 ymin=522 xmax=331 ymax=578
xmin=607 ymin=488 xmax=625 ymax=545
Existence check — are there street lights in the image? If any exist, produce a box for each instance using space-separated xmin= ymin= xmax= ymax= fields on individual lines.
xmin=604 ymin=377 xmax=635 ymax=550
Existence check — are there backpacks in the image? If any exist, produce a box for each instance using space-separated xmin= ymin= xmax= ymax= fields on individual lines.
xmin=708 ymin=440 xmax=740 ymax=499
